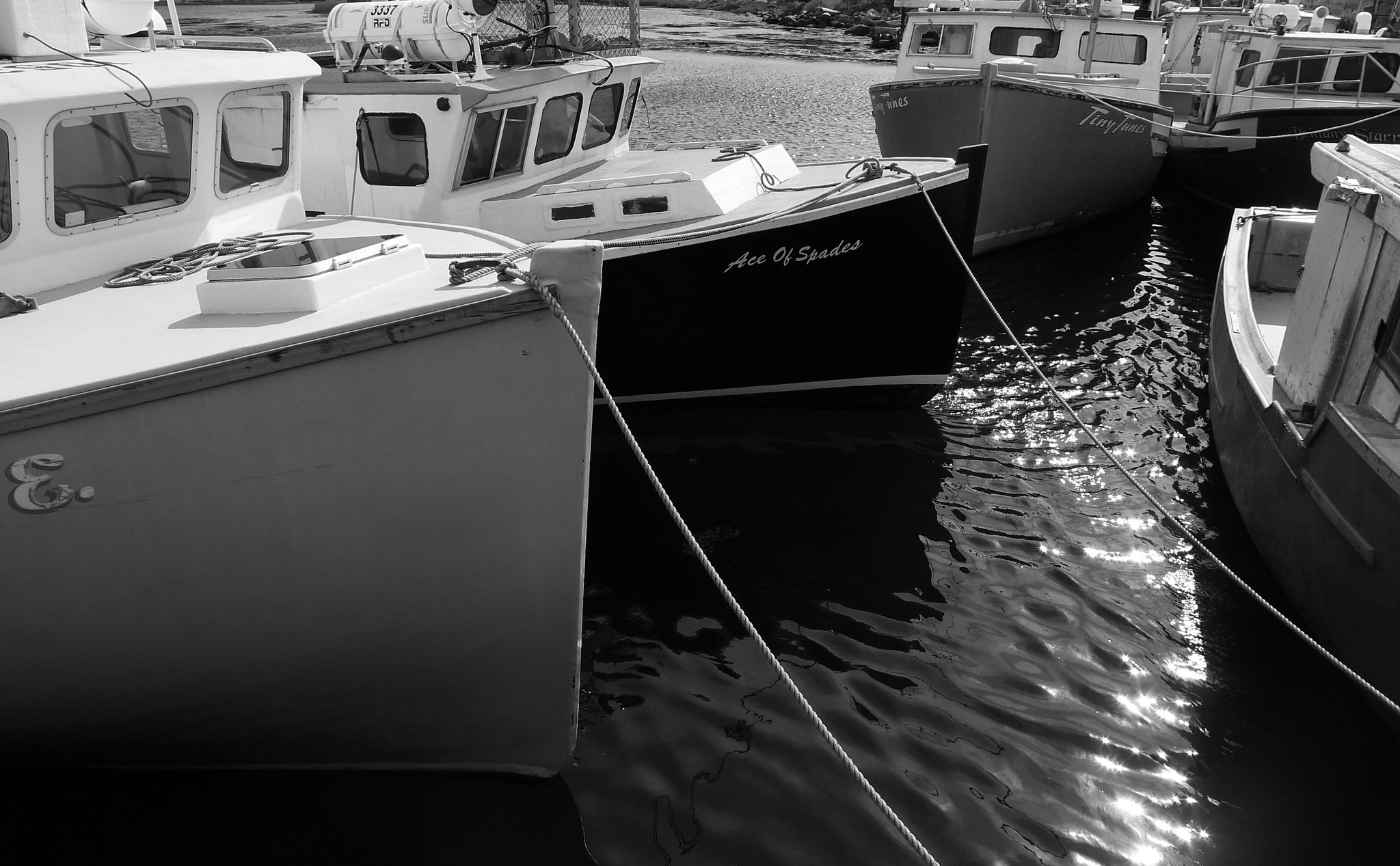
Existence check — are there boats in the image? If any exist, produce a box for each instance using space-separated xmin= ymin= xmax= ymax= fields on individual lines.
xmin=1206 ymin=132 xmax=1399 ymax=706
xmin=868 ymin=1 xmax=1168 ymax=257
xmin=1151 ymin=3 xmax=1400 ymax=204
xmin=0 ymin=2 xmax=608 ymax=778
xmin=295 ymin=4 xmax=989 ymax=408
